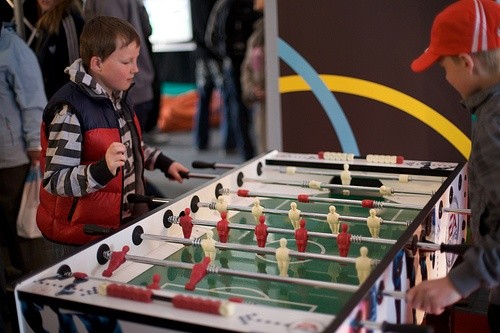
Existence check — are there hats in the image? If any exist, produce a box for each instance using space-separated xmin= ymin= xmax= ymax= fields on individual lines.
xmin=411 ymin=0 xmax=500 ymax=72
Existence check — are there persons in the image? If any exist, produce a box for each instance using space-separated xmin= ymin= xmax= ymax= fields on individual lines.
xmin=34 ymin=1 xmax=161 ymax=134
xmin=35 ymin=17 xmax=190 ymax=253
xmin=410 ymin=0 xmax=500 ymax=333
xmin=0 ymin=1 xmax=52 ymax=293
xmin=188 ymin=0 xmax=266 ymax=162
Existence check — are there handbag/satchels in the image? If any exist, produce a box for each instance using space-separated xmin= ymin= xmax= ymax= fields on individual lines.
xmin=16 ymin=161 xmax=43 ymax=239
